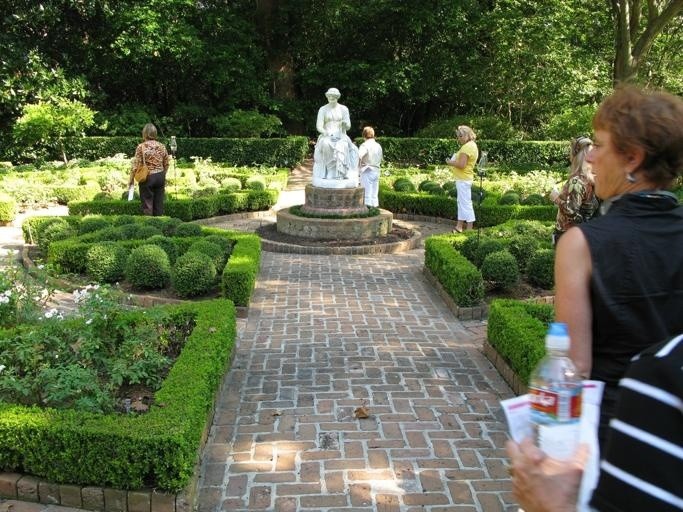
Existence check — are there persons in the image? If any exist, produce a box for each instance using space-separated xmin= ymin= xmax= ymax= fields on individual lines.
xmin=553 ymin=86 xmax=683 ymax=453
xmin=550 ymin=137 xmax=601 ymax=250
xmin=504 ymin=333 xmax=683 ymax=512
xmin=313 ymin=88 xmax=360 ymax=180
xmin=446 ymin=125 xmax=479 ymax=234
xmin=129 ymin=123 xmax=169 ymax=216
xmin=358 ymin=126 xmax=383 ymax=208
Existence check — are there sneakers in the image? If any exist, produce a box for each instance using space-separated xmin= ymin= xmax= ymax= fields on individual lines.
xmin=450 ymin=227 xmax=462 ymax=234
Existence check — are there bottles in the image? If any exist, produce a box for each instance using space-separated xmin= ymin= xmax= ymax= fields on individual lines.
xmin=524 ymin=321 xmax=585 ymax=462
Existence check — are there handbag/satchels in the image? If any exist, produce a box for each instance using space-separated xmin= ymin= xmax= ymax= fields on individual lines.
xmin=133 ymin=165 xmax=148 ymax=184
